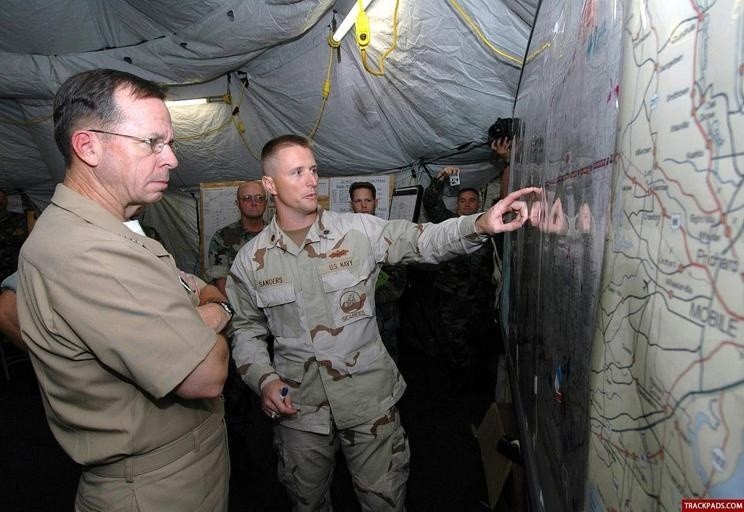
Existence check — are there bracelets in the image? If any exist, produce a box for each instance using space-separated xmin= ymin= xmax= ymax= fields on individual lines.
xmin=210 ymin=299 xmax=233 ymax=336
xmin=503 ymin=160 xmax=510 ymax=169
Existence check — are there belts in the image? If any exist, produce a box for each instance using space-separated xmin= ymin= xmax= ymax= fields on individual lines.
xmin=89 ymin=395 xmax=225 ymax=478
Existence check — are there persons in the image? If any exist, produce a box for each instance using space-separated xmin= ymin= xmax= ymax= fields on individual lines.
xmin=421 ymin=164 xmax=495 ymax=405
xmin=15 ymin=66 xmax=233 ymax=512
xmin=346 ymin=181 xmax=408 ymax=383
xmin=221 ymin=132 xmax=534 ymax=512
xmin=200 ymin=178 xmax=276 ymax=479
xmin=529 ymin=190 xmax=595 ymax=238
xmin=488 ymin=136 xmax=514 ymax=199
xmin=0 ymin=205 xmax=170 ymax=512
xmin=0 ymin=188 xmax=27 ymax=292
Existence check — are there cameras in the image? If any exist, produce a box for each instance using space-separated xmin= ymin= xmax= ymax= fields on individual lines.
xmin=489 ymin=117 xmax=513 ymax=142
xmin=450 ymin=171 xmax=460 ymax=186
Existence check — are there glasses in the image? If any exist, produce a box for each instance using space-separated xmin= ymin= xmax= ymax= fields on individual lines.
xmin=90 ymin=127 xmax=178 ymax=154
xmin=239 ymin=194 xmax=265 ymax=203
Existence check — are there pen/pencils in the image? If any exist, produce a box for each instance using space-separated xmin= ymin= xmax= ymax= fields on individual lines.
xmin=272 ymin=387 xmax=288 ymax=418
